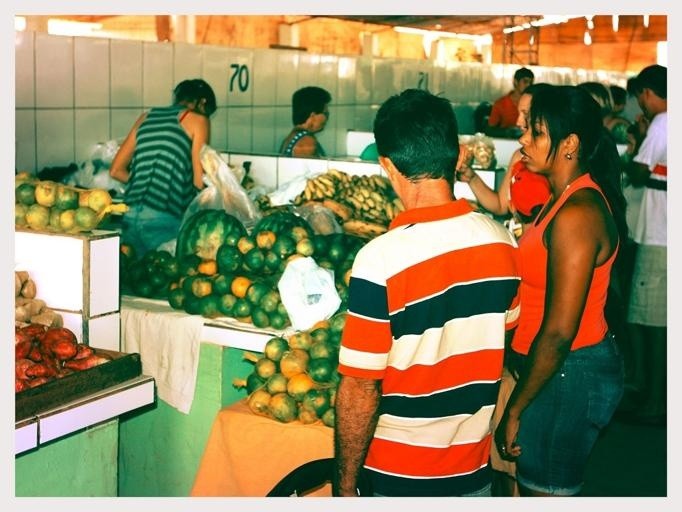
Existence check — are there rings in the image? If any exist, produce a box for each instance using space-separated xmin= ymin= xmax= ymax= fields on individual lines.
xmin=500 ymin=446 xmax=506 ymax=451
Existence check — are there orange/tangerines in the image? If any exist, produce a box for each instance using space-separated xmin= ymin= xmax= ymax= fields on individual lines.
xmin=14 ymin=181 xmax=369 ymax=427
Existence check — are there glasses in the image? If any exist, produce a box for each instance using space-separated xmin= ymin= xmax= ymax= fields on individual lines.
xmin=322 ymin=111 xmax=330 ymax=118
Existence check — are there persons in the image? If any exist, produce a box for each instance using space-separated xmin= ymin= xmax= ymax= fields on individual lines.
xmin=504 ymin=160 xmax=554 ymax=241
xmin=326 ymin=88 xmax=521 ymax=495
xmin=494 ymin=88 xmax=627 ymax=495
xmin=275 ymin=85 xmax=333 ymax=158
xmin=610 ymin=64 xmax=666 ymax=425
xmin=484 ymin=66 xmax=651 ymax=162
xmin=105 ymin=77 xmax=217 ymax=256
xmin=457 ymin=84 xmax=549 ymax=216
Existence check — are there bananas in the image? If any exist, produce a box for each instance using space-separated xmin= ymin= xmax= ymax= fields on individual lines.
xmin=296 ymin=168 xmax=406 ymax=223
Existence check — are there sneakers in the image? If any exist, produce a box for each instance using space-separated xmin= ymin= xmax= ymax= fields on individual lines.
xmin=612 ymin=405 xmax=666 ymax=426
xmin=622 ymin=382 xmax=642 ymax=396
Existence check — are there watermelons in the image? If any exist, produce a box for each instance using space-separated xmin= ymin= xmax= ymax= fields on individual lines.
xmin=175 ymin=209 xmax=314 ymax=260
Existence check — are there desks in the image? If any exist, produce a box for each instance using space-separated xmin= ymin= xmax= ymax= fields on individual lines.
xmin=189 ymin=395 xmax=336 ymax=496
